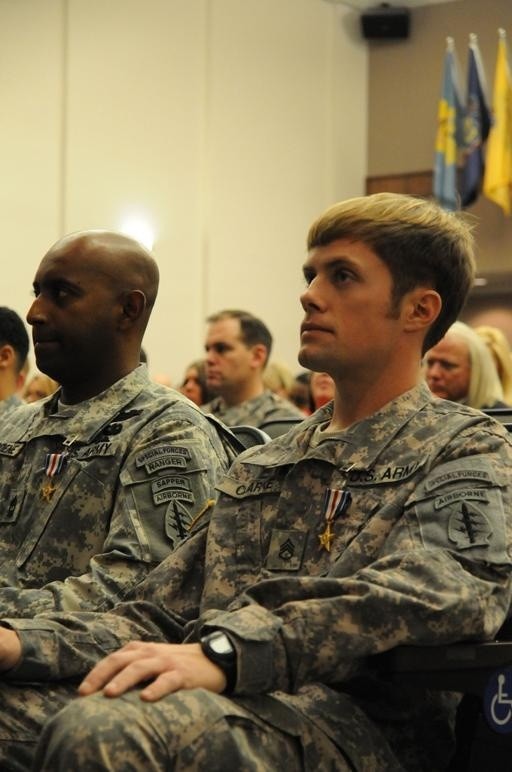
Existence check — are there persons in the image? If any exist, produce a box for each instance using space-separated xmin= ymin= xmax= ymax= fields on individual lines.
xmin=179 ymin=310 xmax=339 ymax=443
xmin=0 ymin=229 xmax=227 ymax=771
xmin=420 ymin=319 xmax=511 ymax=411
xmin=1 ymin=193 xmax=512 ymax=772
xmin=1 ymin=308 xmax=59 ymax=419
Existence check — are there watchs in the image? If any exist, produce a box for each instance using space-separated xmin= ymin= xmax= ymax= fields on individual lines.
xmin=199 ymin=629 xmax=237 ymax=697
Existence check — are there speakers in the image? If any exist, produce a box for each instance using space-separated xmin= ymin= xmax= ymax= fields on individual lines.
xmin=360 ymin=8 xmax=410 ymax=40
